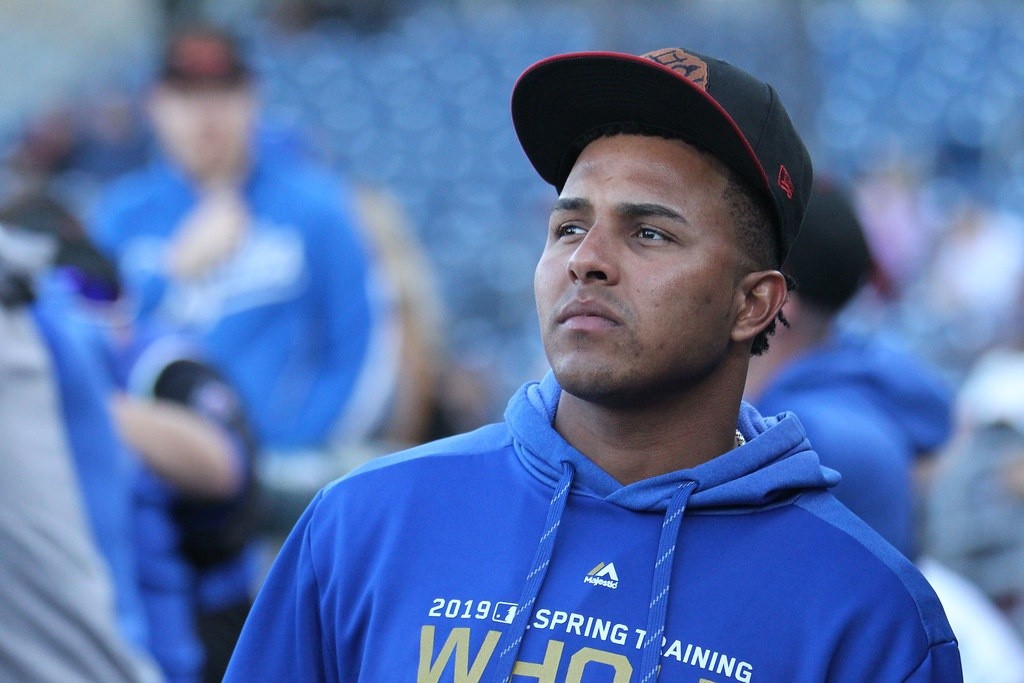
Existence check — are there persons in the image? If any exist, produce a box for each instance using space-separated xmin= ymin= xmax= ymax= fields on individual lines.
xmin=832 ymin=141 xmax=1024 ymax=616
xmin=741 ymin=174 xmax=954 ymax=565
xmin=221 ymin=44 xmax=961 ymax=681
xmin=82 ymin=30 xmax=404 ymax=488
xmin=1 ymin=197 xmax=262 ymax=681
xmin=1 ymin=251 xmax=163 ymax=681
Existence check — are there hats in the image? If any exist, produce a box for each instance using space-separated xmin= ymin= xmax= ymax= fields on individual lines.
xmin=156 ymin=16 xmax=255 ymax=91
xmin=509 ymin=46 xmax=812 ymax=257
xmin=763 ymin=177 xmax=872 ymax=320
